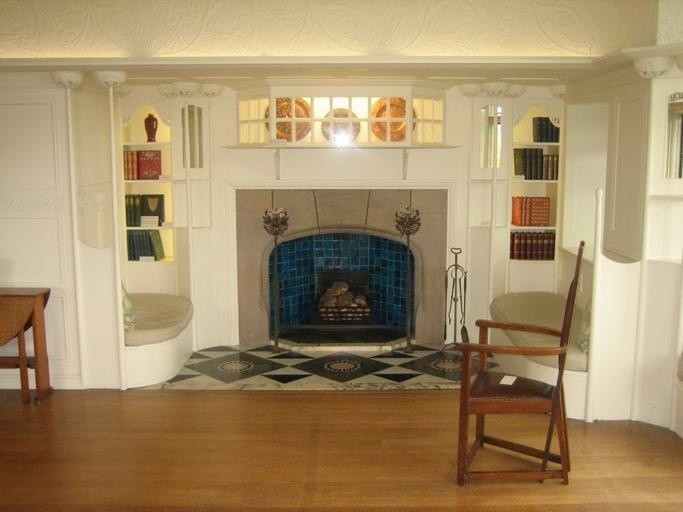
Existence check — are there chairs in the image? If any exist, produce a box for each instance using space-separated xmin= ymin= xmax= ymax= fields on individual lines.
xmin=454 ymin=240 xmax=585 ymax=486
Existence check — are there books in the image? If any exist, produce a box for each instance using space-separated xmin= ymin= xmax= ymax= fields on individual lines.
xmin=513 ymin=147 xmax=558 ymax=181
xmin=509 ymin=196 xmax=555 ymax=262
xmin=125 ymin=193 xmax=166 ymax=261
xmin=123 ymin=149 xmax=162 ymax=180
xmin=532 ymin=116 xmax=560 ymax=142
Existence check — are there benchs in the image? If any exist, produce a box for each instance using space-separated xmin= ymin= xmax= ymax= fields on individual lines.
xmin=491 ymin=291 xmax=586 ymax=423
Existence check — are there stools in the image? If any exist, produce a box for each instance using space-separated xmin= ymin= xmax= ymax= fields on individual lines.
xmin=121 ymin=288 xmax=195 ymax=390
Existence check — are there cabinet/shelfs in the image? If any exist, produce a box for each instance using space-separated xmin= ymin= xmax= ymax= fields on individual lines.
xmin=506 ymin=85 xmax=568 ymax=293
xmin=602 ymin=79 xmax=682 ymax=260
xmin=123 ymin=84 xmax=178 ymax=297
